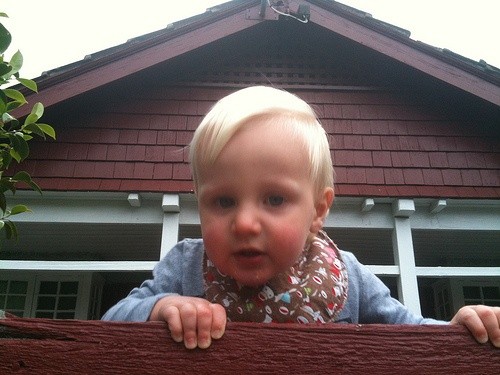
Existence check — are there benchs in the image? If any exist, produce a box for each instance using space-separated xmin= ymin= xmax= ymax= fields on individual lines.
xmin=0 ymin=311 xmax=500 ymax=375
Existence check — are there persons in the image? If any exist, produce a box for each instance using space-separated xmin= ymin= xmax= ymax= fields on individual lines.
xmin=98 ymin=85 xmax=499 ymax=348
